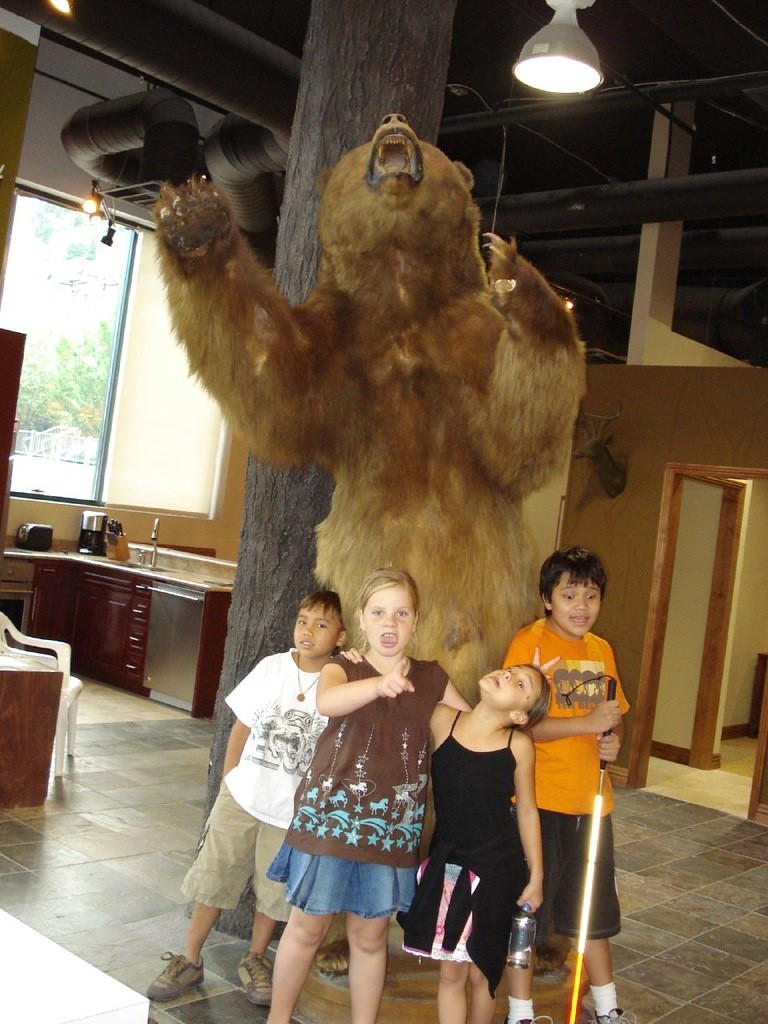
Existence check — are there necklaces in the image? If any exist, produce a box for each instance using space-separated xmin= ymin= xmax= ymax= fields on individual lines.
xmin=297 ymin=654 xmax=321 ymax=702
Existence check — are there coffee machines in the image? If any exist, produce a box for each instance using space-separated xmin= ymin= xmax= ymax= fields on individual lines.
xmin=76 ymin=510 xmax=107 ymax=556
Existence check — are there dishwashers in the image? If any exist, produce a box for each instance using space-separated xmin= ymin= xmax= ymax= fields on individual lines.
xmin=143 ymin=581 xmax=205 ymax=703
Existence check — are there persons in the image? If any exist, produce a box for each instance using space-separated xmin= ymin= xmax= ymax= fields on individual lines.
xmin=264 ymin=569 xmax=473 ymax=1024
xmin=148 ymin=588 xmax=344 ymax=1006
xmin=340 ymin=646 xmax=554 ymax=1024
xmin=504 ymin=548 xmax=630 ymax=1024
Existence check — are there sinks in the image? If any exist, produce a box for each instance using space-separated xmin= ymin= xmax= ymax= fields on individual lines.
xmin=94 ymin=560 xmax=175 ymax=572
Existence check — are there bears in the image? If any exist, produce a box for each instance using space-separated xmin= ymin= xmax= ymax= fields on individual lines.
xmin=153 ymin=110 xmax=586 ymax=981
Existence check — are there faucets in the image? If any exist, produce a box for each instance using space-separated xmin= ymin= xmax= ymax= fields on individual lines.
xmin=149 ymin=518 xmax=160 ymax=568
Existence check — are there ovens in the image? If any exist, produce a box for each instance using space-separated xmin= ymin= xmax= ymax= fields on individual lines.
xmin=0 ymin=583 xmax=35 ymax=652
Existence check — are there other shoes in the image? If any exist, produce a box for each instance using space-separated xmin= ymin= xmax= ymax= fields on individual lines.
xmin=503 ymin=1016 xmax=553 ymax=1024
xmin=588 ymin=1008 xmax=638 ymax=1024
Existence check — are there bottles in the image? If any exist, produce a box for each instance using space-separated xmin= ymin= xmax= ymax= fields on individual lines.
xmin=506 ymin=903 xmax=537 ymax=969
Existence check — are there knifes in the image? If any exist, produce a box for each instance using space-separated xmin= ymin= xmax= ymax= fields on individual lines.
xmin=105 ymin=519 xmax=124 ymax=545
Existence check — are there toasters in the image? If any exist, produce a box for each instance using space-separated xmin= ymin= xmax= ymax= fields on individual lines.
xmin=16 ymin=523 xmax=53 ymax=550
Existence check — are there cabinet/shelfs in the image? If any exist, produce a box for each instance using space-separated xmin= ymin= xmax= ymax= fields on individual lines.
xmin=26 ymin=560 xmax=153 ymax=700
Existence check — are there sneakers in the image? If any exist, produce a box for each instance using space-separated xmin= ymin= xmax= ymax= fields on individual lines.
xmin=237 ymin=951 xmax=272 ymax=1006
xmin=147 ymin=951 xmax=204 ymax=1002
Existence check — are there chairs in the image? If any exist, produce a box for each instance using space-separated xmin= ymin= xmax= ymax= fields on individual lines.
xmin=0 ymin=611 xmax=85 ymax=778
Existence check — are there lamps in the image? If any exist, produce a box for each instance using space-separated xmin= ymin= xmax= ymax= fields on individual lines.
xmin=513 ymin=0 xmax=605 ymax=94
xmin=101 ymin=218 xmax=116 ymax=247
